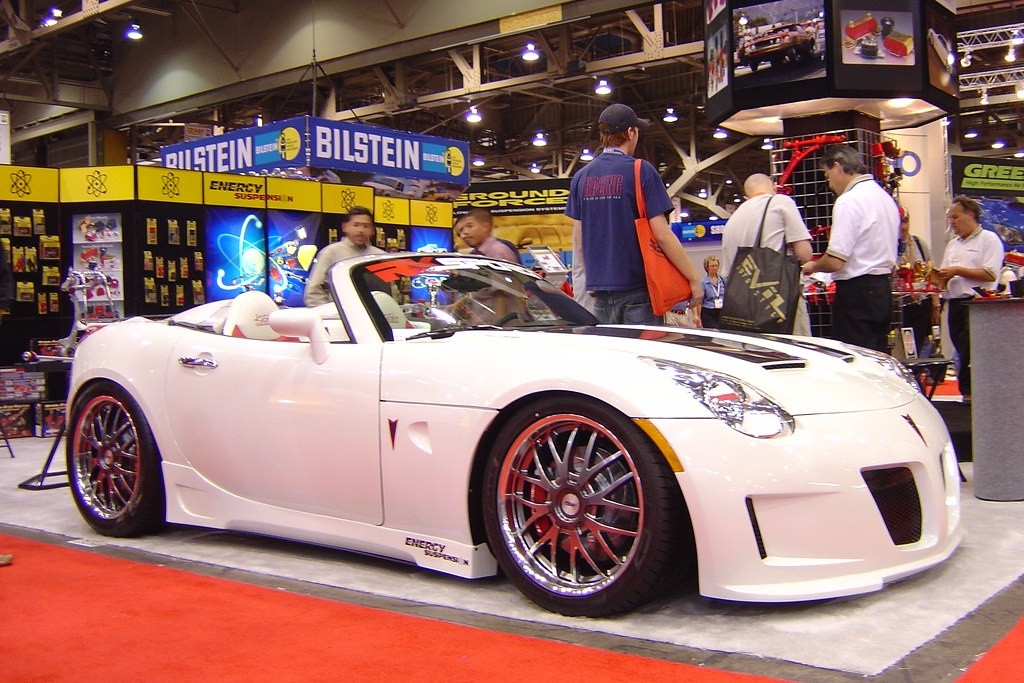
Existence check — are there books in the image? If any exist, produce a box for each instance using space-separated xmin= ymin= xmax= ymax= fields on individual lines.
xmin=928 ymin=267 xmax=955 ymax=290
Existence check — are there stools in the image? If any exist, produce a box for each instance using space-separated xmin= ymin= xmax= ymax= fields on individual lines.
xmin=899 ymin=356 xmax=968 ymax=482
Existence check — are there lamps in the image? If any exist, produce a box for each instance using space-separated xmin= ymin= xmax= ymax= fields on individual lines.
xmin=761 ymin=138 xmax=774 ymax=150
xmin=980 ymin=93 xmax=989 ymax=105
xmin=530 ymin=162 xmax=540 ymax=174
xmin=532 ymin=132 xmax=547 ymax=146
xmin=466 ymin=106 xmax=482 ymax=123
xmin=663 ymin=107 xmax=678 ymax=122
xmin=522 ymin=42 xmax=540 ymax=61
xmin=1005 ymin=48 xmax=1017 ymax=62
xmin=960 ymin=54 xmax=971 ymax=67
xmin=126 ymin=17 xmax=144 ymax=40
xmin=595 ymin=79 xmax=612 ymax=95
xmin=580 ymin=148 xmax=594 ymax=161
xmin=713 ymin=129 xmax=728 ymax=139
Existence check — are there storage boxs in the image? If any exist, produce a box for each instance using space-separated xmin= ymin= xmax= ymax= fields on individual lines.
xmin=0 ymin=371 xmax=47 ymax=402
xmin=0 ymin=207 xmax=205 ymax=315
xmin=0 ymin=403 xmax=36 ymax=440
xmin=34 ymin=399 xmax=67 ymax=438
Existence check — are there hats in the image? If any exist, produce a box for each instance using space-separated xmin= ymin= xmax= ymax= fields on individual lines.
xmin=598 ymin=104 xmax=649 ymax=135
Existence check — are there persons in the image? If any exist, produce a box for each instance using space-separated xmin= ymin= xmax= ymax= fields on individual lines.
xmin=304 ymin=207 xmax=399 ymax=306
xmin=895 ymin=207 xmax=940 ymax=386
xmin=708 ymin=41 xmax=724 ymax=92
xmin=717 ymin=173 xmax=814 ymax=337
xmin=564 ymin=103 xmax=705 ymax=327
xmin=701 ymin=256 xmax=728 ymax=329
xmin=801 ymin=143 xmax=901 ymax=357
xmin=997 ymin=260 xmax=1017 ymax=295
xmin=443 ymin=208 xmax=529 ymax=328
xmin=938 ymin=194 xmax=1004 ymax=402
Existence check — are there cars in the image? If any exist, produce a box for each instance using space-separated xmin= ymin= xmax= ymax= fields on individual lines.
xmin=62 ymin=251 xmax=964 ymax=619
xmin=743 ymin=22 xmax=816 ymax=72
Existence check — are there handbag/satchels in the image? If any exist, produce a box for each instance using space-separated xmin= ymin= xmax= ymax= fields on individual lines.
xmin=722 ymin=194 xmax=802 ymax=335
xmin=633 ymin=158 xmax=693 ymax=315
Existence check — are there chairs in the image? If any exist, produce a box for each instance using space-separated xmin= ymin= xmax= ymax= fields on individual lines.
xmin=222 ymin=290 xmax=306 ymax=342
xmin=370 ymin=290 xmax=416 ymax=330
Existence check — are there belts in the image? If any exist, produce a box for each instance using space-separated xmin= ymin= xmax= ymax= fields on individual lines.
xmin=670 ymin=310 xmax=686 ymax=314
